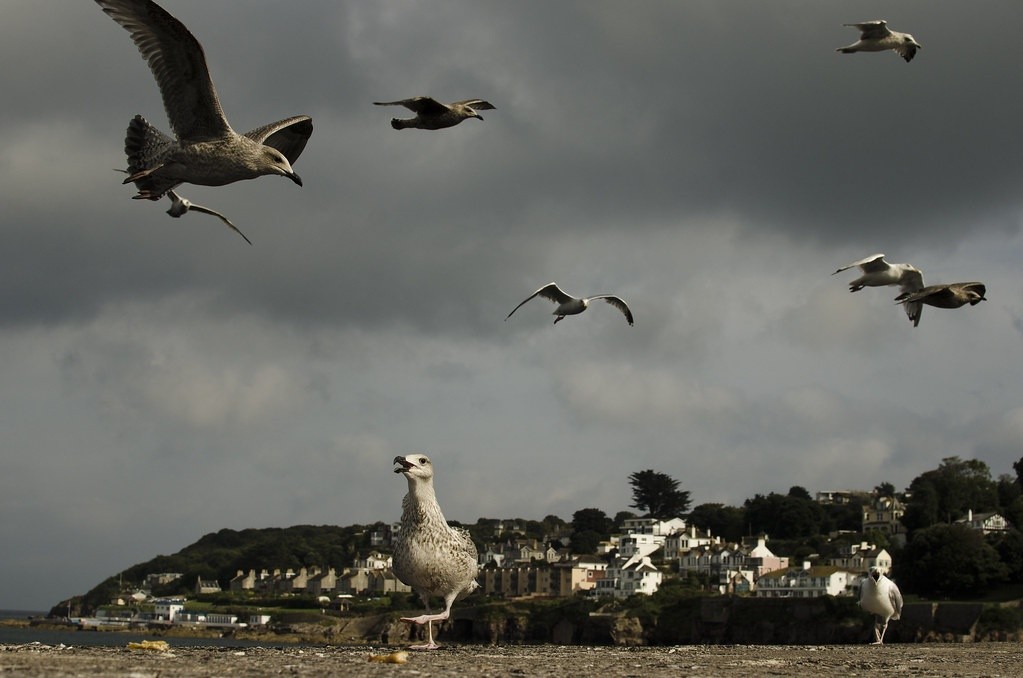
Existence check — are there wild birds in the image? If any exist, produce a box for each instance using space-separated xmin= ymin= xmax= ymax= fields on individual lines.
xmin=390 ymin=453 xmax=482 ymax=650
xmin=503 ymin=282 xmax=637 ymax=328
xmin=373 ymin=95 xmax=497 ymax=131
xmin=834 ymin=20 xmax=923 ymax=63
xmin=114 ymin=168 xmax=253 ymax=249
xmin=827 ymin=253 xmax=921 ymax=291
xmin=856 ymin=566 xmax=904 ymax=645
xmin=92 ymin=0 xmax=314 ymax=201
xmin=895 ymin=268 xmax=989 ymax=327
